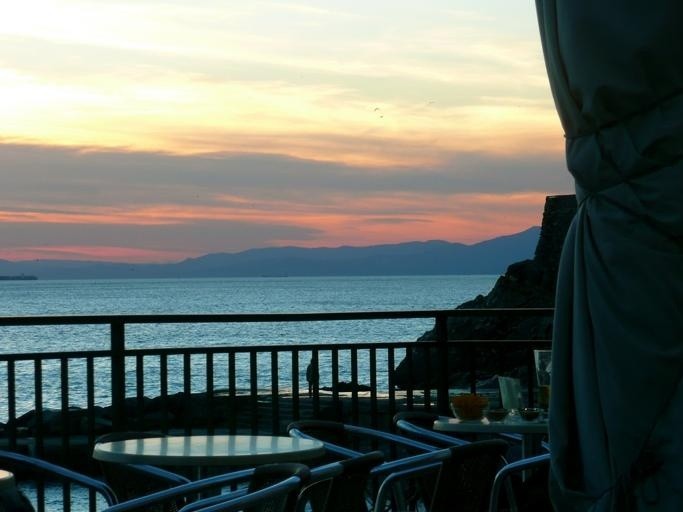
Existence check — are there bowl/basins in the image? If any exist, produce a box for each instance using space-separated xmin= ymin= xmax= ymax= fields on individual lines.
xmin=519 ymin=407 xmax=540 ymax=420
xmin=449 ymin=393 xmax=489 ymax=424
xmin=482 ymin=407 xmax=509 ymax=423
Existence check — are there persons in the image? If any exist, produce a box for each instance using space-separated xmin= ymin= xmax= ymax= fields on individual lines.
xmin=305 ymin=358 xmax=315 ymax=399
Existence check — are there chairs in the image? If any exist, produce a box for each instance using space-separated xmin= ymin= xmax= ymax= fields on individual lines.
xmin=0 ymin=406 xmax=550 ymax=512
